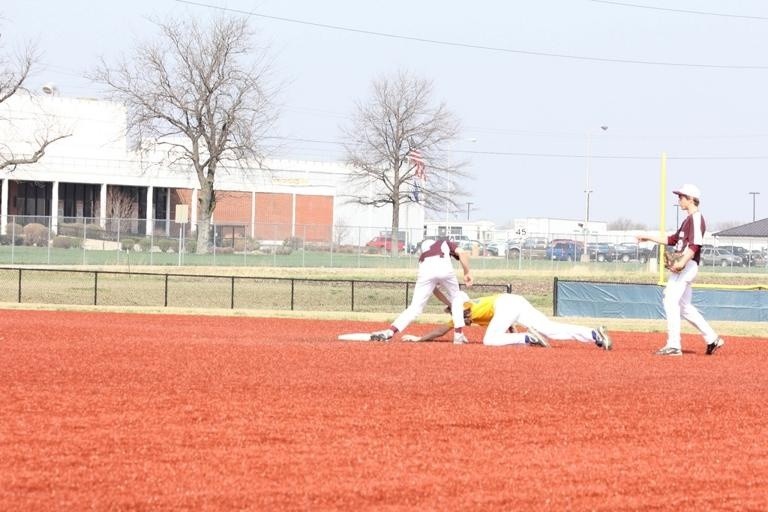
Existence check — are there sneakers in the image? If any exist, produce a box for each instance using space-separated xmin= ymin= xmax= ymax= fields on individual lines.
xmin=525 ymin=326 xmax=551 ymax=348
xmin=653 ymin=343 xmax=684 ymax=357
xmin=370 ymin=328 xmax=395 ymax=342
xmin=593 ymin=325 xmax=613 ymax=350
xmin=703 ymin=336 xmax=725 ymax=356
xmin=453 ymin=330 xmax=469 ymax=344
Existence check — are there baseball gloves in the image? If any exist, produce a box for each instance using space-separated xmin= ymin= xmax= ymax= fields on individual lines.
xmin=663 ymin=250 xmax=685 ymax=273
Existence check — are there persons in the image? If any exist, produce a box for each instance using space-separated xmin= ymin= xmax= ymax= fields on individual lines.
xmin=637 ymin=183 xmax=725 ymax=355
xmin=370 ymin=237 xmax=471 ymax=345
xmin=402 ymin=293 xmax=614 ymax=354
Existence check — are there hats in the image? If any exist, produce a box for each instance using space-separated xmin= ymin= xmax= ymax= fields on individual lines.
xmin=672 ymin=181 xmax=701 ymax=203
xmin=444 ymin=291 xmax=470 ymax=313
xmin=420 ymin=238 xmax=436 ymax=254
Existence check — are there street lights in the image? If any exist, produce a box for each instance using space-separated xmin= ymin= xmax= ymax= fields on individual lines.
xmin=579 ymin=124 xmax=609 ymax=262
xmin=746 ymin=190 xmax=760 ymax=221
xmin=462 ymin=200 xmax=474 ymax=222
xmin=670 ymin=202 xmax=681 ymax=232
xmin=444 ymin=135 xmax=478 ymax=238
xmin=584 ymin=190 xmax=593 ymax=222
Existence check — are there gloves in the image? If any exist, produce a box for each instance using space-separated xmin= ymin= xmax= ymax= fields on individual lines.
xmin=401 ymin=334 xmax=423 ymax=343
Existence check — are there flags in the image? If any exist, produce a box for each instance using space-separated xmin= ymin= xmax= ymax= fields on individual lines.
xmin=403 ymin=137 xmax=427 ymax=182
